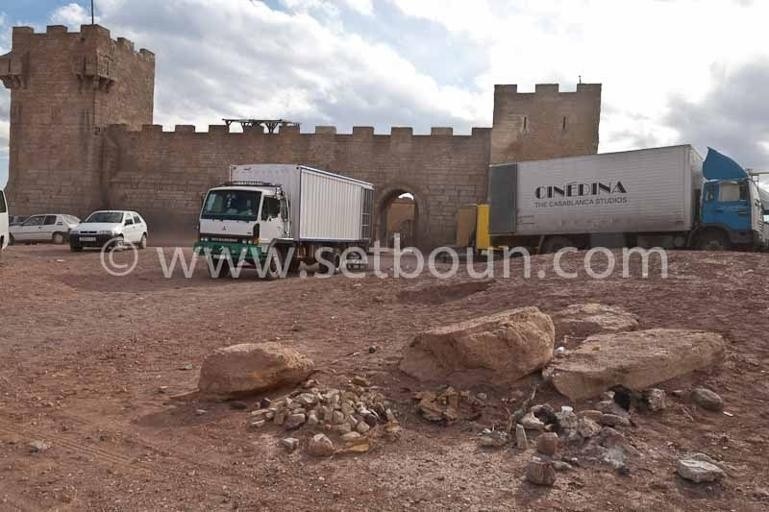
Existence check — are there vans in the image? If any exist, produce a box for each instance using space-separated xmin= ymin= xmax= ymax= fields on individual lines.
xmin=0 ymin=189 xmax=13 ymax=255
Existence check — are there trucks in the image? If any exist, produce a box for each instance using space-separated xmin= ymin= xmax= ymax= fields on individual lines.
xmin=479 ymin=143 xmax=767 ymax=255
xmin=193 ymin=164 xmax=374 ymax=278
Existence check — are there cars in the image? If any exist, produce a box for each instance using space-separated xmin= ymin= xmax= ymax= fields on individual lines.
xmin=8 ymin=213 xmax=83 ymax=245
xmin=69 ymin=209 xmax=150 ymax=249
